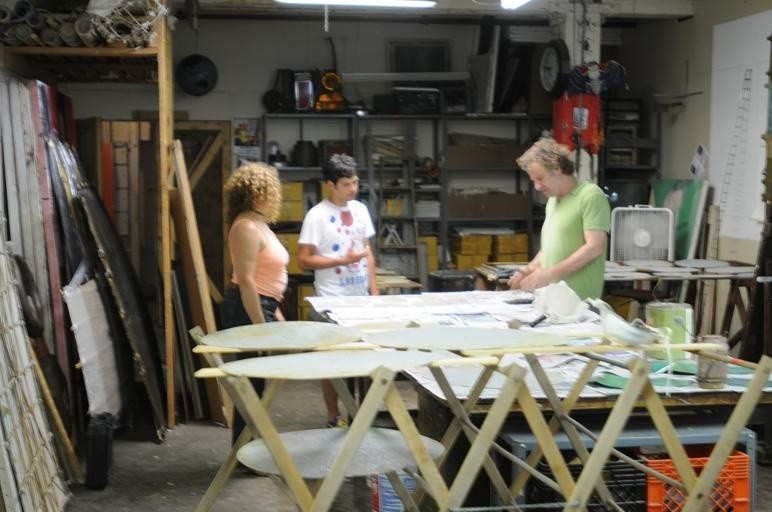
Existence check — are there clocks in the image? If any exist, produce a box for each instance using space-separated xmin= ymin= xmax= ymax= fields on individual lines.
xmin=538 ymin=38 xmax=570 ymax=96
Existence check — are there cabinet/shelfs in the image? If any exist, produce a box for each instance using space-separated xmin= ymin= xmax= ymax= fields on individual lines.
xmin=185 ymin=320 xmax=449 ymax=511
xmin=261 ymin=106 xmax=664 ymax=318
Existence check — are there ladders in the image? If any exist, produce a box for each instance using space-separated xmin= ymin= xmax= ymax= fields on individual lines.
xmin=375 ymin=155 xmax=425 ymax=295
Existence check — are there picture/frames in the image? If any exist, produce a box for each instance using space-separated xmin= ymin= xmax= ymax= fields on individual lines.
xmin=388 ymin=39 xmax=454 ymax=88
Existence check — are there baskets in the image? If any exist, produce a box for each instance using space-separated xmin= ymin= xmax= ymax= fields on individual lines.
xmin=524 ymin=447 xmax=647 ymax=512
xmin=630 ymin=443 xmax=750 ymax=512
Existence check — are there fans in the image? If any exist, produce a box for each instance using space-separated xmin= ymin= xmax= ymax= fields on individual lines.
xmin=609 ymin=204 xmax=675 ymax=267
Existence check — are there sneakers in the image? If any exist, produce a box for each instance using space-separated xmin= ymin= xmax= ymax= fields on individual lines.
xmin=326 ymin=416 xmax=349 ymax=428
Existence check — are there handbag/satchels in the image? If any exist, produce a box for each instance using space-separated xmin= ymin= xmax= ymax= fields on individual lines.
xmin=85 ymin=413 xmax=119 ymax=488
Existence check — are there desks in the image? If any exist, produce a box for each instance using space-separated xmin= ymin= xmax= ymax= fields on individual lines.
xmin=471 ymin=257 xmax=758 ymax=362
xmin=299 ymin=288 xmax=770 ymax=512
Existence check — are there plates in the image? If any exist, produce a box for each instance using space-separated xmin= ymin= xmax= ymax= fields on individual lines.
xmin=603 ymin=256 xmax=755 ymax=279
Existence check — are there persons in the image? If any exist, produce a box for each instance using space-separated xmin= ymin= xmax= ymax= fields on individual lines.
xmin=217 ymin=161 xmax=290 ymax=478
xmin=508 ymin=136 xmax=612 ymax=309
xmin=297 ymin=154 xmax=380 ymax=427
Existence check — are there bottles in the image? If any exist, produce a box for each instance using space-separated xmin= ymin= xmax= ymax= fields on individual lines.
xmin=697 ymin=335 xmax=732 ymax=389
xmin=268 ymin=140 xmax=280 ymax=164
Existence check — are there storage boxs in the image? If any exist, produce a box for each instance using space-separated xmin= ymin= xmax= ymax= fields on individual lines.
xmin=276 ymin=181 xmax=530 ymax=279
